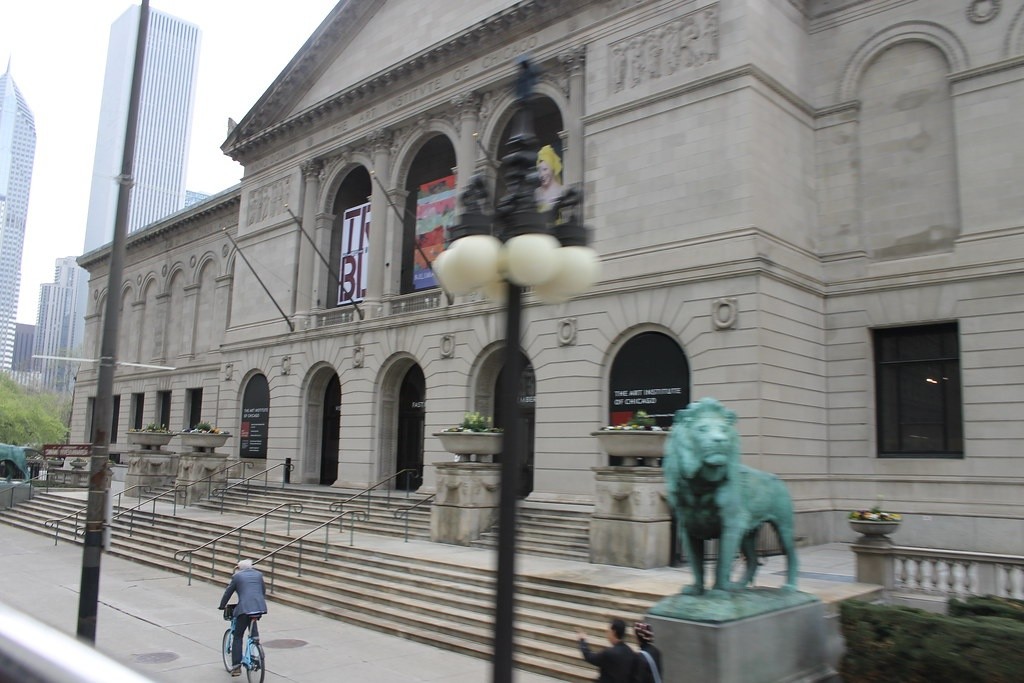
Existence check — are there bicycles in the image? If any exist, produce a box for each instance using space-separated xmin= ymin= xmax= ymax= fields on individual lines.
xmin=217 ymin=603 xmax=266 ymax=683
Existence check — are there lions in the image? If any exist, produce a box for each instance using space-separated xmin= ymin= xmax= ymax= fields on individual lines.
xmin=662 ymin=398 xmax=800 ymax=596
xmin=0 ymin=442 xmax=44 ymax=483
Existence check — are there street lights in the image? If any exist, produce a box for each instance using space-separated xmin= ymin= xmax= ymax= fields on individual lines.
xmin=430 ymin=233 xmax=602 ymax=683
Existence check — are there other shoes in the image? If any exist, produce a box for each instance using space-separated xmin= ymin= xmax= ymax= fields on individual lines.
xmin=231 ymin=665 xmax=240 ymax=676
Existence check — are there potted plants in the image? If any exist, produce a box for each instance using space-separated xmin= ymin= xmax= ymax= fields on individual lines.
xmin=47 ymin=456 xmax=64 ymax=469
xmin=69 ymin=457 xmax=88 ymax=470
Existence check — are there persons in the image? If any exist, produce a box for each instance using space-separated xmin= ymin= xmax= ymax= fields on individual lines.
xmin=220 ymin=559 xmax=268 ymax=676
xmin=634 ymin=620 xmax=663 ymax=683
xmin=579 ymin=618 xmax=640 ymax=683
xmin=532 ymin=144 xmax=562 ymax=212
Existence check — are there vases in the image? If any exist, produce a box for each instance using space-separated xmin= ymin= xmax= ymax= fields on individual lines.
xmin=591 ymin=431 xmax=667 ymax=473
xmin=847 ymin=521 xmax=901 ymax=545
xmin=432 ymin=431 xmax=504 ymax=469
xmin=125 ymin=432 xmax=178 ymax=453
xmin=177 ymin=433 xmax=233 ymax=457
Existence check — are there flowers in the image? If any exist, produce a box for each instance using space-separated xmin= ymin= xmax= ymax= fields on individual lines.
xmin=183 ymin=421 xmax=230 ymax=435
xmin=600 ymin=409 xmax=674 ymax=432
xmin=130 ymin=423 xmax=173 ymax=434
xmin=440 ymin=413 xmax=501 ymax=433
xmin=848 ymin=495 xmax=901 ymax=522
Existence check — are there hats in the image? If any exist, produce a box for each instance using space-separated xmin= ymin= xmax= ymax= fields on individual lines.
xmin=634 ymin=622 xmax=655 ymax=641
